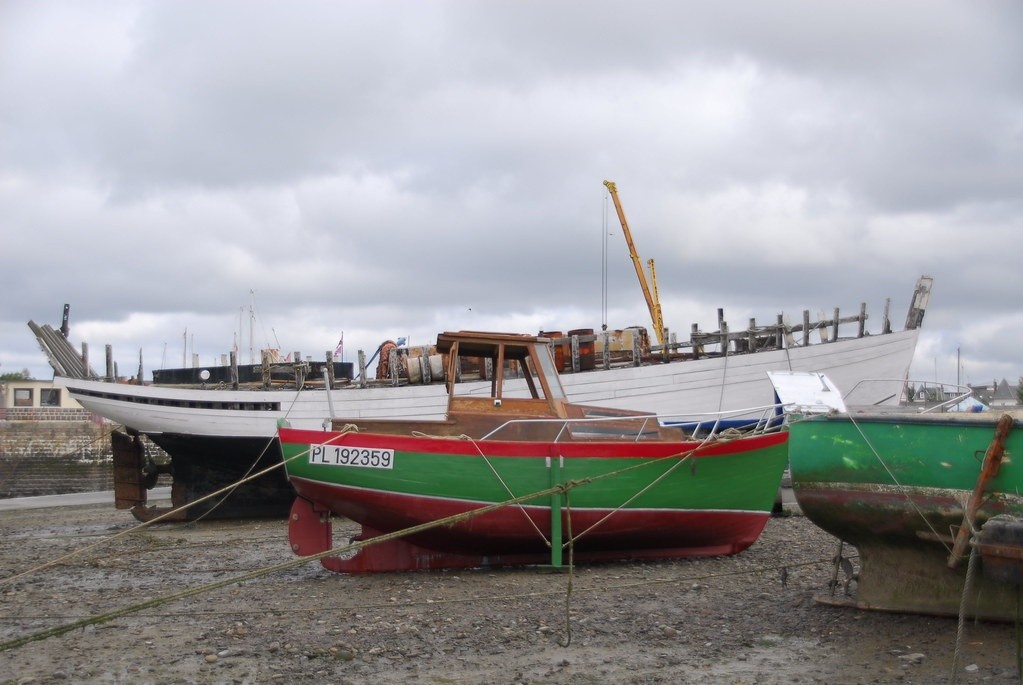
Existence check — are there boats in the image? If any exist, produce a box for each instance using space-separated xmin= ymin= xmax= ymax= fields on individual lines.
xmin=273 ymin=328 xmax=801 ymax=576
xmin=27 ymin=176 xmax=937 ymax=526
xmin=785 ymin=378 xmax=1023 ymax=629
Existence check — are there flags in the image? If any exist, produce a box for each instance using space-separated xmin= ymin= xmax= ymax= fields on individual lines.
xmin=335 ymin=333 xmax=343 ymax=354
